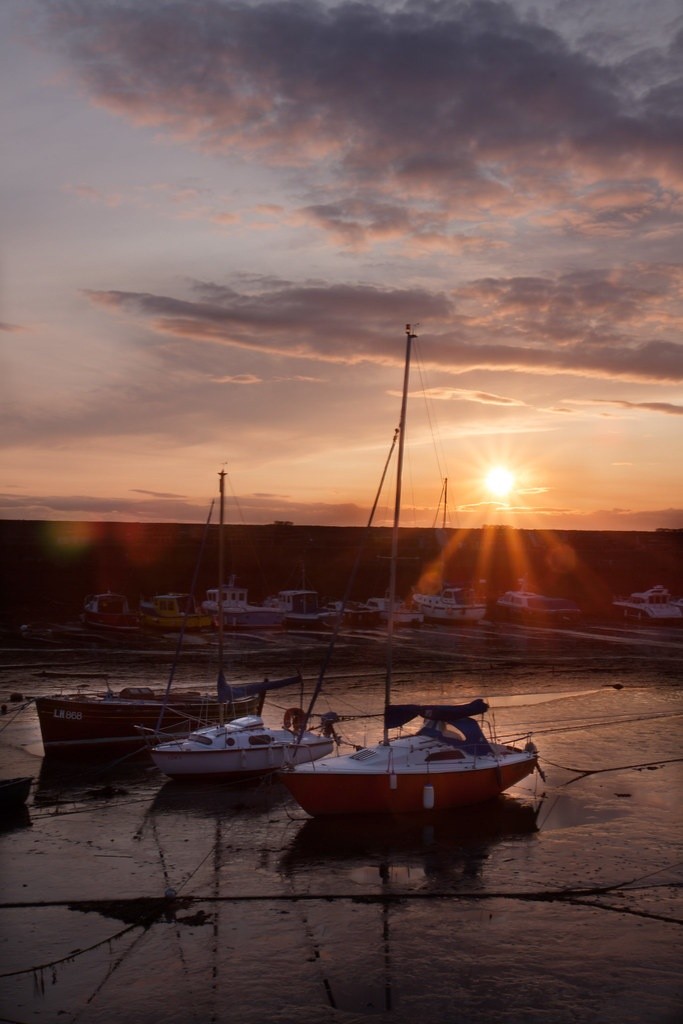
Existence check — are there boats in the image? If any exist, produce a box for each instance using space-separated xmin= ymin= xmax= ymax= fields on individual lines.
xmin=318 ymin=596 xmax=384 ymax=631
xmin=34 ymin=680 xmax=267 ymax=769
xmin=80 ymin=591 xmax=146 ymax=632
xmin=136 ymin=592 xmax=212 ymax=632
xmin=264 ymin=590 xmax=344 ymax=630
xmin=495 ymin=572 xmax=582 ymax=629
xmin=356 ymin=598 xmax=424 ymax=627
xmin=610 ymin=584 xmax=683 ymax=622
xmin=200 ymin=574 xmax=287 ymax=630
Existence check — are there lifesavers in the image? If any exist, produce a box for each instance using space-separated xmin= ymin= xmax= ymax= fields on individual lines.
xmin=283 ymin=708 xmax=307 ymax=729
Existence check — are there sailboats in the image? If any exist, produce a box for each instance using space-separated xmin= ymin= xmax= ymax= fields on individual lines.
xmin=150 ymin=468 xmax=335 ymax=783
xmin=413 ymin=478 xmax=487 ymax=624
xmin=276 ymin=324 xmax=538 ymax=820
